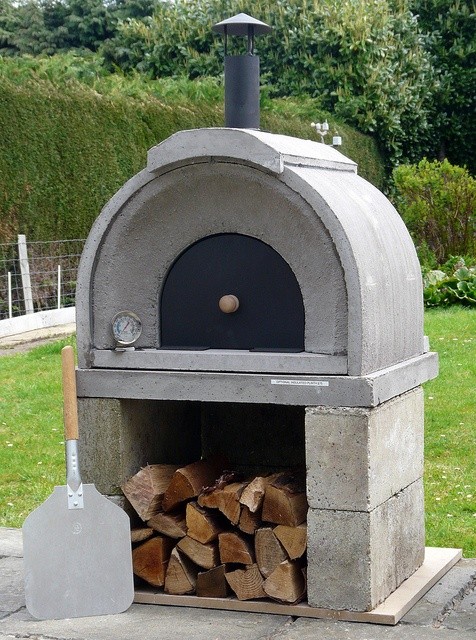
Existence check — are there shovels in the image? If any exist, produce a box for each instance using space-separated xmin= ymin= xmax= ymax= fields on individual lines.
xmin=22 ymin=345 xmax=135 ymax=620
